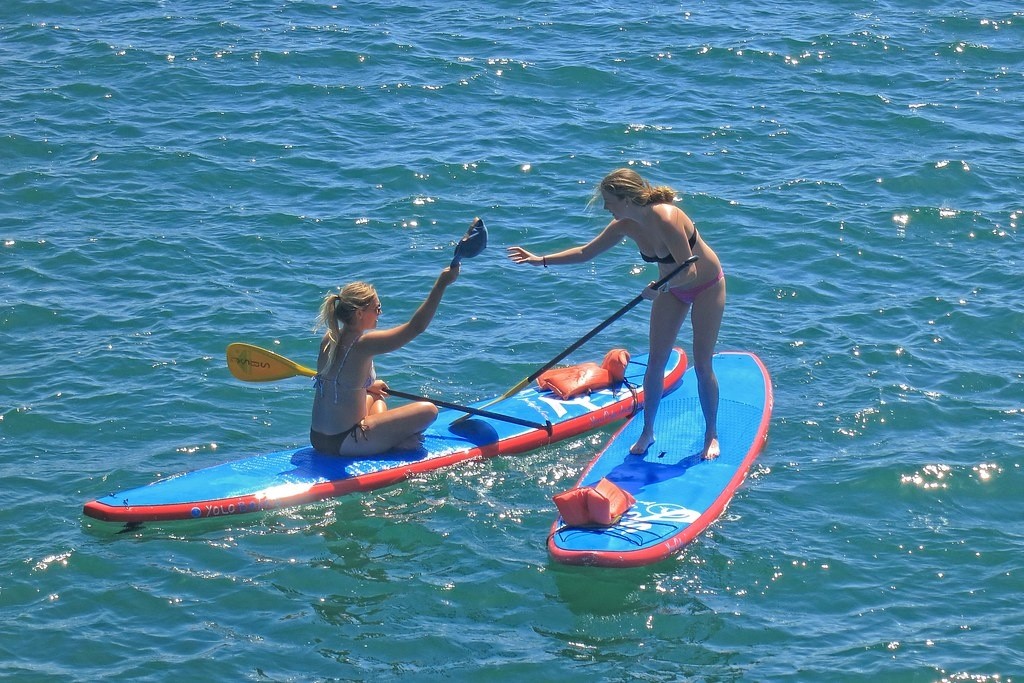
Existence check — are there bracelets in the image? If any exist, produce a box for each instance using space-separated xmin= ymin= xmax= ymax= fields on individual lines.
xmin=541 ymin=255 xmax=547 ymax=266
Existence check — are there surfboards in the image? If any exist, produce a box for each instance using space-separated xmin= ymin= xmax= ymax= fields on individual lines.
xmin=82 ymin=345 xmax=688 ymax=523
xmin=548 ymin=350 xmax=773 ymax=568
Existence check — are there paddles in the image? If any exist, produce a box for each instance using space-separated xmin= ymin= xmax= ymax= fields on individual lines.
xmin=448 ymin=255 xmax=700 ymax=426
xmin=225 ymin=343 xmax=553 ymax=437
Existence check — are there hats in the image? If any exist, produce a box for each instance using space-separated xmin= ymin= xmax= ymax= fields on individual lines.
xmin=450 ymin=217 xmax=488 ymax=269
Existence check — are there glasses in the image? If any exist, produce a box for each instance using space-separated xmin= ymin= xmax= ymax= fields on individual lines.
xmin=362 ymin=303 xmax=382 ymax=314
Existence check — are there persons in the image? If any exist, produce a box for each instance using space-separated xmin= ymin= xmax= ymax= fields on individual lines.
xmin=505 ymin=169 xmax=725 ymax=460
xmin=310 ymin=263 xmax=460 ymax=459
